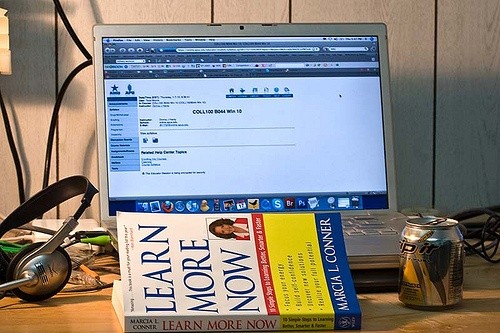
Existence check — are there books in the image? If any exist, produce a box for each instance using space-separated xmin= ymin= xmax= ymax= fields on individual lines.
xmin=110 ymin=210 xmax=362 ymax=333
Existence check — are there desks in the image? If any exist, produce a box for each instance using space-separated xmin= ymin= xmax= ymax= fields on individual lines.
xmin=0 ymin=215 xmax=499 ymax=333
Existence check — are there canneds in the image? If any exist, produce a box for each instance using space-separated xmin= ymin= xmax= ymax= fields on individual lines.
xmin=400 ymin=217 xmax=464 ymax=306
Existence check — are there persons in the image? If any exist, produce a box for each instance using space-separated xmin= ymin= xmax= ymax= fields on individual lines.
xmin=209 ymin=218 xmax=250 ymax=240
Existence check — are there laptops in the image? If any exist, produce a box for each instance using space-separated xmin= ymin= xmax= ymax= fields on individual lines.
xmin=93 ymin=23 xmax=410 ymax=271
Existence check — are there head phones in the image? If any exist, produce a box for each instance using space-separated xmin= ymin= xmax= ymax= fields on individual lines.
xmin=0 ymin=176 xmax=98 ymax=302
